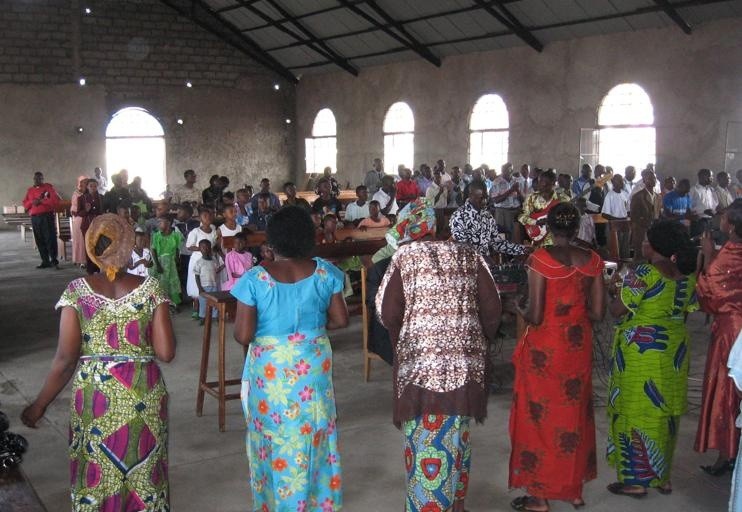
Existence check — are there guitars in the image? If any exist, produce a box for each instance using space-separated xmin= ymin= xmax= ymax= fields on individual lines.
xmin=525 ymin=173 xmax=614 ymax=244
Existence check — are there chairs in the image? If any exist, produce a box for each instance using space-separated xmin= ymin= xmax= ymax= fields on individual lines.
xmin=608 ymin=219 xmax=634 ymax=267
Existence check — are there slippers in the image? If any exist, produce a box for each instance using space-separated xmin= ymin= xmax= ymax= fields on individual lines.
xmin=607 ymin=482 xmax=647 ymax=499
xmin=510 ymin=495 xmax=549 ymax=512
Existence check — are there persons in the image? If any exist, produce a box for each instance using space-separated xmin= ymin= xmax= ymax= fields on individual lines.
xmin=604 ymin=221 xmax=695 ymax=496
xmin=68 ymin=155 xmax=742 ymax=329
xmin=724 ymin=329 xmax=742 ymax=512
xmin=375 ymin=201 xmax=501 ymax=512
xmin=21 ymin=213 xmax=176 ymax=512
xmin=230 ymin=206 xmax=349 ymax=512
xmin=694 ymin=198 xmax=742 ymax=476
xmin=22 ymin=171 xmax=59 ymax=269
xmin=507 ymin=202 xmax=605 ymax=511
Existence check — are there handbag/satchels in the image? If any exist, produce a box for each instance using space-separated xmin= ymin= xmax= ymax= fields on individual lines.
xmin=337 ymin=255 xmax=367 ymax=272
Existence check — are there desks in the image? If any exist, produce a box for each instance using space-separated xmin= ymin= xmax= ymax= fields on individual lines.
xmin=196 ymin=191 xmax=391 ymax=432
xmin=589 ymin=213 xmax=608 ymax=224
xmin=2 ymin=206 xmax=71 ymax=261
xmin=491 ymin=254 xmax=529 ymax=340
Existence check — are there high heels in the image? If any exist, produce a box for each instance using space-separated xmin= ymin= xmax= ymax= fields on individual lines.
xmin=700 ymin=458 xmax=736 ymax=480
